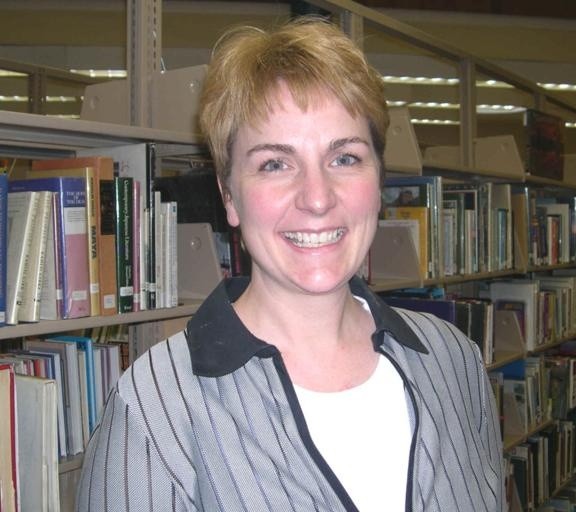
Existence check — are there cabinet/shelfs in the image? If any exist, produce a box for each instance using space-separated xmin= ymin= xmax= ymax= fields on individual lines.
xmin=1 ymin=1 xmax=576 ymax=511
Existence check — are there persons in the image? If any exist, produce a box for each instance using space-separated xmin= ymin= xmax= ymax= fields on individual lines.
xmin=74 ymin=16 xmax=505 ymax=512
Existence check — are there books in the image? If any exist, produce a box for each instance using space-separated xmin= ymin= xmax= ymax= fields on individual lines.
xmin=505 ymin=416 xmax=576 ymax=512
xmin=526 ymin=109 xmax=565 ymax=181
xmin=486 ymin=353 xmax=576 ymax=444
xmin=0 ymin=142 xmax=178 ymax=326
xmin=0 ymin=316 xmax=192 ymax=512
xmin=154 ymin=164 xmax=241 ymax=277
xmin=375 ymin=276 xmax=576 ymax=364
xmin=358 ymin=177 xmax=575 ymax=283
